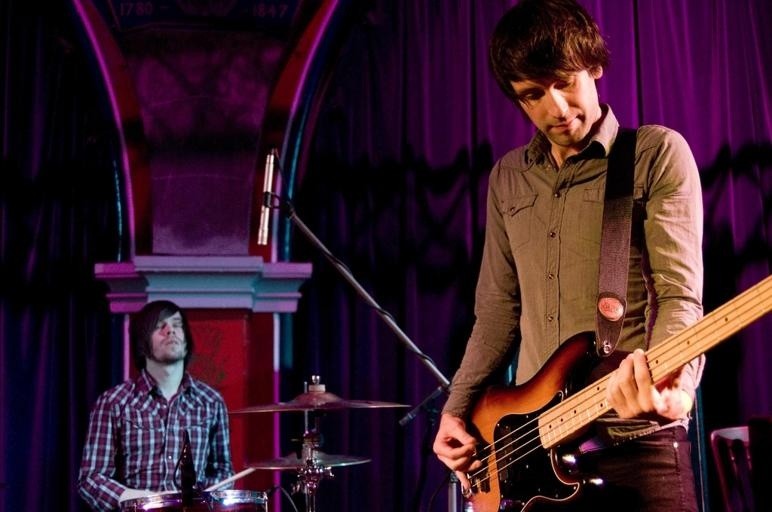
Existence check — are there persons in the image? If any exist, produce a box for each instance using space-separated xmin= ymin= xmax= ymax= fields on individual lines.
xmin=74 ymin=299 xmax=236 ymax=512
xmin=432 ymin=0 xmax=709 ymax=511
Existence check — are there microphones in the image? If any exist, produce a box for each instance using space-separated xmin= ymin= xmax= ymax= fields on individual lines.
xmin=257 ymin=150 xmax=275 ymax=246
xmin=182 ymin=429 xmax=197 ymax=487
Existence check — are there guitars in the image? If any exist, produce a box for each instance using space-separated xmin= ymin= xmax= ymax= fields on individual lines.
xmin=465 ymin=275 xmax=771 ymax=511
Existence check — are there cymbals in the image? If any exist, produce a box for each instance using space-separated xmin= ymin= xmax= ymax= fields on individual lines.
xmin=251 ymin=450 xmax=370 ymax=469
xmin=227 ymin=390 xmax=413 ymax=413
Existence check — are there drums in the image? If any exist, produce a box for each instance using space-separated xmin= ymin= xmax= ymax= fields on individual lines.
xmin=122 ymin=489 xmax=270 ymax=512
